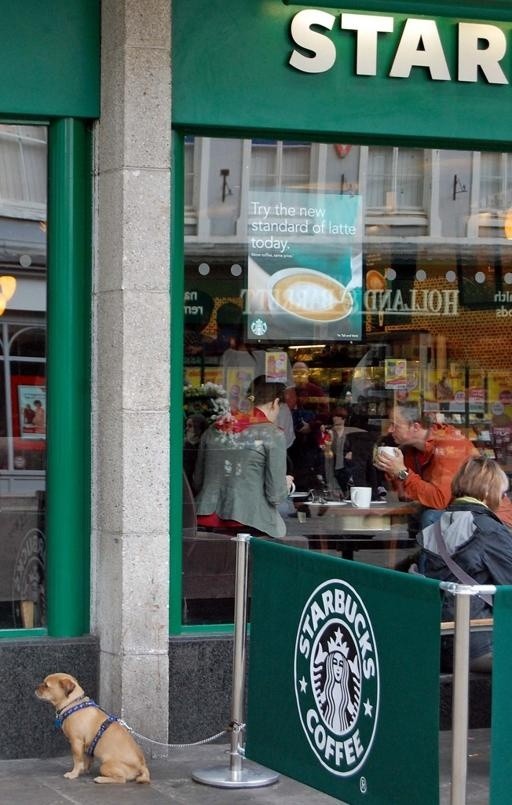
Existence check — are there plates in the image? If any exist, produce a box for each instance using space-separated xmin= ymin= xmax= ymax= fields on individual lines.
xmin=303 ymin=501 xmax=347 ymax=507
xmin=345 ymin=500 xmax=387 ymax=505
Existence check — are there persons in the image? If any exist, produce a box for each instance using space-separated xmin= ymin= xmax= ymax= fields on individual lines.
xmin=202 ymin=301 xmax=244 ymax=358
xmin=24 ymin=403 xmax=35 ymax=423
xmin=182 ymin=414 xmax=210 ymax=487
xmin=369 ymin=397 xmax=511 ymax=532
xmin=31 ymin=400 xmax=46 ymax=427
xmin=184 ymin=289 xmax=220 ymax=357
xmin=409 ymin=454 xmax=510 ymax=674
xmin=281 ymin=360 xmax=331 ymax=491
xmin=186 ymin=373 xmax=299 ymax=536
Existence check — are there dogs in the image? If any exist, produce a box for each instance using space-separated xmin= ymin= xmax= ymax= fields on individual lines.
xmin=32 ymin=671 xmax=151 ymax=786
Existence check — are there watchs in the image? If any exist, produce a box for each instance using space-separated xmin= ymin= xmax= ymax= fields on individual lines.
xmin=397 ymin=468 xmax=409 ymax=481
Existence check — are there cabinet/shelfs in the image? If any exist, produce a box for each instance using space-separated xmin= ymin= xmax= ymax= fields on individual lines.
xmin=286 ymin=329 xmax=444 ymax=432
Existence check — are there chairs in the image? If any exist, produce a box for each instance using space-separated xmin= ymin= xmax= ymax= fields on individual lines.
xmin=182 ymin=470 xmax=309 ymax=623
xmin=0 ymin=496 xmax=50 ymax=631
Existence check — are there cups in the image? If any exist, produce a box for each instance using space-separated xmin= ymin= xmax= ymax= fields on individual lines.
xmin=264 ymin=267 xmax=354 ymax=338
xmin=286 ymin=475 xmax=295 ymax=495
xmin=378 ymin=447 xmax=398 ymax=460
xmin=350 ymin=486 xmax=372 ymax=508
